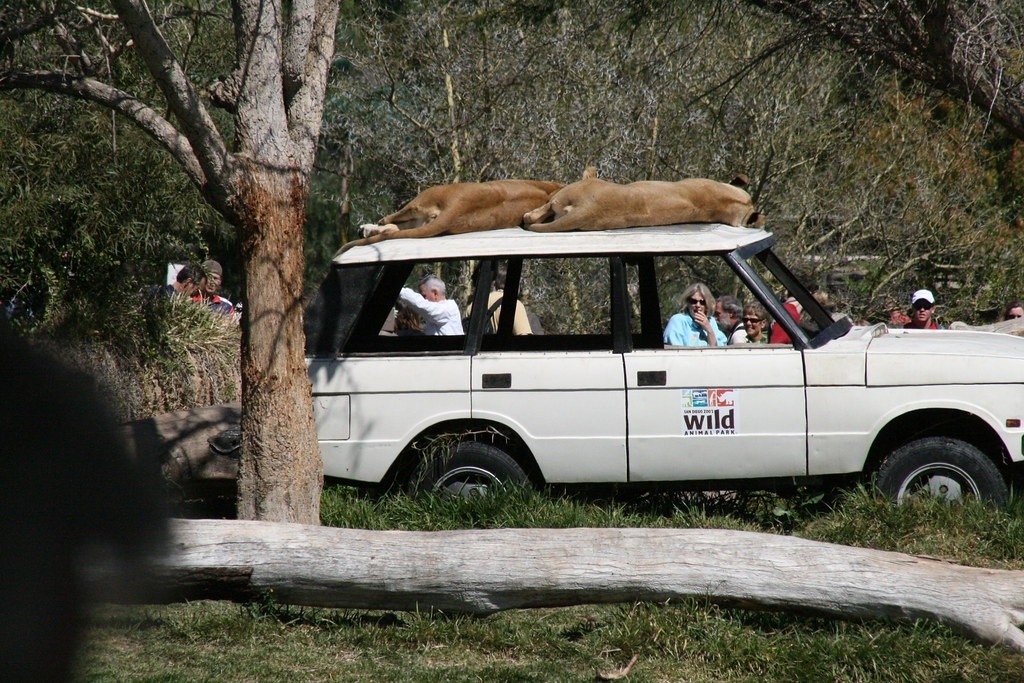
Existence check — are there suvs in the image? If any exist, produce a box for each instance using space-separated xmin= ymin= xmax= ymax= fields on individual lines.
xmin=302 ymin=221 xmax=1024 ymax=525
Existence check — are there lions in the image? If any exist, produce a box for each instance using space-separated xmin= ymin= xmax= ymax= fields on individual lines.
xmin=327 ymin=166 xmax=764 ymax=262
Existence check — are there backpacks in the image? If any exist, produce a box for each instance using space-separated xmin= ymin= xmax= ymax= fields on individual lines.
xmin=462 ymin=298 xmax=503 ymax=335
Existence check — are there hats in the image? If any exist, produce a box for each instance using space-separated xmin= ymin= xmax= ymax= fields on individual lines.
xmin=911 ymin=289 xmax=935 ymax=305
xmin=201 ymin=260 xmax=223 ymax=276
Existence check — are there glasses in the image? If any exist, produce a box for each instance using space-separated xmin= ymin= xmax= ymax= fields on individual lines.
xmin=741 ymin=318 xmax=764 ymax=323
xmin=687 ymin=296 xmax=706 ymax=305
xmin=913 ymin=302 xmax=934 ymax=311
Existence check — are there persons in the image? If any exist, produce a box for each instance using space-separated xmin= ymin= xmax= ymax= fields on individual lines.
xmin=166 ymin=264 xmax=208 ymax=301
xmin=191 ymin=260 xmax=234 ymax=321
xmin=903 ymin=289 xmax=946 ymax=330
xmin=1005 ymin=301 xmax=1024 ymax=321
xmin=390 ymin=263 xmax=546 ymax=336
xmin=664 ymin=282 xmax=870 ymax=348
xmin=0 ymin=316 xmax=172 ymax=683
